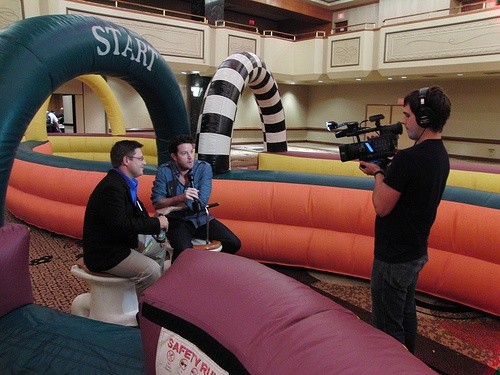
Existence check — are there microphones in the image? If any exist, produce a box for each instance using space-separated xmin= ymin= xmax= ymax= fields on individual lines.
xmin=187 ymin=174 xmax=201 ymax=213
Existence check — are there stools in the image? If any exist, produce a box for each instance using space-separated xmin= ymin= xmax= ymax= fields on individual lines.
xmin=69 ymin=264 xmax=139 ymax=328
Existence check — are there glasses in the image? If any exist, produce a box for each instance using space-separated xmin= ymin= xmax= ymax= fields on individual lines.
xmin=129 ymin=156 xmax=143 ymax=161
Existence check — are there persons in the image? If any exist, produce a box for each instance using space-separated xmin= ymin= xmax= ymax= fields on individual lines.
xmin=82 ymin=140 xmax=169 ymax=301
xmin=46 ymin=108 xmax=64 ymax=133
xmin=359 ymin=86 xmax=451 ymax=355
xmin=150 ymin=135 xmax=241 ymax=267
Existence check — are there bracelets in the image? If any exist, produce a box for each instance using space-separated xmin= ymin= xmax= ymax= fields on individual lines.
xmin=374 ymin=170 xmax=385 ymax=176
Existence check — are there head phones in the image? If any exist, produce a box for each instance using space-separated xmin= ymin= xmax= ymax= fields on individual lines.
xmin=416 ymin=87 xmax=434 ymax=128
